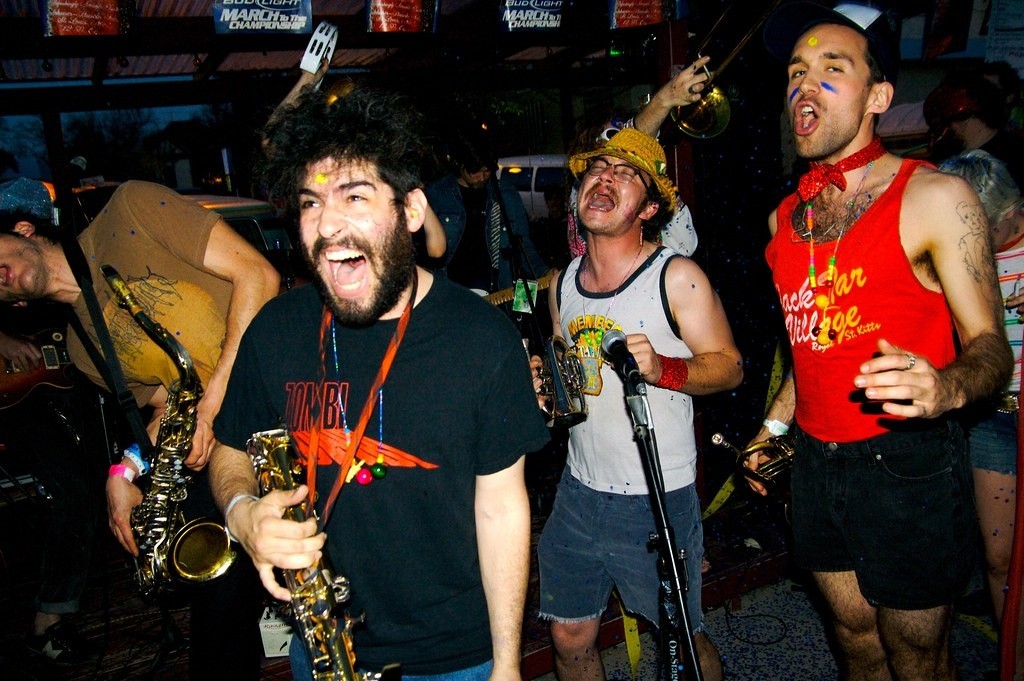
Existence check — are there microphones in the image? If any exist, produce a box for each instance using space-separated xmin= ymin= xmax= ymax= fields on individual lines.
xmin=601 ymin=330 xmax=647 ymax=395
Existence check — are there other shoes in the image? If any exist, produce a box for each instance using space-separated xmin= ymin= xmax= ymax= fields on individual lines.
xmin=21 ymin=617 xmax=94 ymax=666
xmin=725 ymin=491 xmax=762 ymax=537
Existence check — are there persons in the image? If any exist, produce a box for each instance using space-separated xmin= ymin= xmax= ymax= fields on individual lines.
xmin=0 ymin=179 xmax=281 ymax=681
xmin=567 ymin=56 xmax=717 ymax=269
xmin=264 ymin=19 xmax=543 ymax=302
xmin=742 ymin=22 xmax=1014 ymax=681
xmin=922 ymin=59 xmax=1024 ymax=630
xmin=529 ymin=127 xmax=744 ymax=681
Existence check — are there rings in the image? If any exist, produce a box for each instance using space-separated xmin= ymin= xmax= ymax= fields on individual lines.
xmin=907 ymin=354 xmax=917 ymax=370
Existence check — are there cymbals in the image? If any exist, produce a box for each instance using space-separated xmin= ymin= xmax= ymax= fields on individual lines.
xmin=299 ymin=20 xmax=339 ymax=94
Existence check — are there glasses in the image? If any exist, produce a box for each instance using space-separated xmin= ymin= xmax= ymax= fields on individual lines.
xmin=477 ymin=158 xmax=498 ymax=168
xmin=586 ymin=158 xmax=653 ymax=202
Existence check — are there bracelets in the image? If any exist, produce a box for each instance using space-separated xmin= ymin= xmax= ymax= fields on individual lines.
xmin=110 ymin=464 xmax=136 ymax=483
xmin=763 ymin=418 xmax=791 ymax=437
xmin=657 ymin=354 xmax=689 ymax=392
xmin=224 ymin=492 xmax=263 ymax=543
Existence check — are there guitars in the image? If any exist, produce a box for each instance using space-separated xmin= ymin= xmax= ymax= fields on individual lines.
xmin=0 ymin=327 xmax=75 ymax=410
xmin=468 ymin=274 xmax=551 ymax=306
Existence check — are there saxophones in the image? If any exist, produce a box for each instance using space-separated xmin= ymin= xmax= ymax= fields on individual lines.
xmin=98 ymin=264 xmax=243 ymax=603
xmin=246 ymin=421 xmax=403 ymax=681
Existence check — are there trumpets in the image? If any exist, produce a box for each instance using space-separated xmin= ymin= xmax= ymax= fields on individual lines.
xmin=711 ymin=432 xmax=795 ymax=527
xmin=522 ymin=334 xmax=589 ymax=430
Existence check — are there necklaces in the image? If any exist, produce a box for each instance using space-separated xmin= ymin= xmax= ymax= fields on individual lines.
xmin=805 ymin=158 xmax=872 ymax=345
xmin=331 ymin=313 xmax=387 ymax=485
xmin=582 ymin=240 xmax=644 ymax=359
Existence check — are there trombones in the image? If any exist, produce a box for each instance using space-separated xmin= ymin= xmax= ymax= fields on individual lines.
xmin=637 ymin=0 xmax=784 ymax=153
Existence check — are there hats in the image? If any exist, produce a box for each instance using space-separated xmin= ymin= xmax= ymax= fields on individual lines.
xmin=762 ymin=0 xmax=902 ymax=91
xmin=923 ymin=66 xmax=1010 ymax=131
xmin=462 ymin=144 xmax=498 ymax=163
xmin=567 ymin=127 xmax=675 ymax=212
